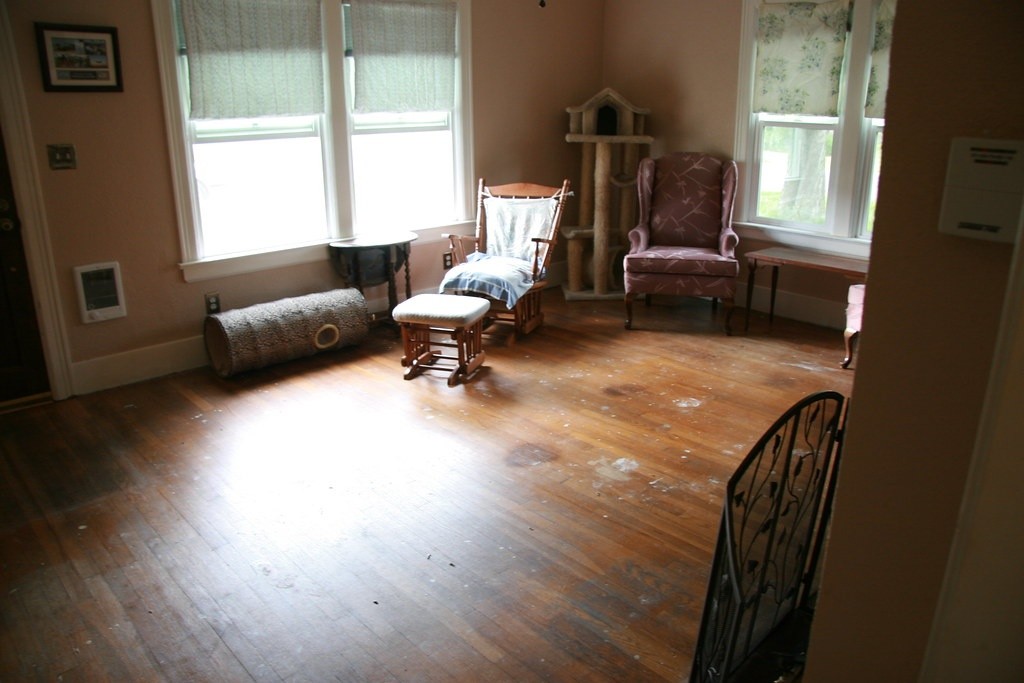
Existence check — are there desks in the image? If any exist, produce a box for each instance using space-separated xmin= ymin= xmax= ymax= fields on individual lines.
xmin=743 ymin=247 xmax=871 ymax=335
xmin=329 ymin=232 xmax=419 ymax=331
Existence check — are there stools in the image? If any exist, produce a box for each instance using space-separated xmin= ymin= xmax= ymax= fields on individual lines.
xmin=392 ymin=294 xmax=491 ymax=386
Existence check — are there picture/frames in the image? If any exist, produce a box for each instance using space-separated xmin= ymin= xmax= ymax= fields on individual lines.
xmin=36 ymin=22 xmax=124 ymax=94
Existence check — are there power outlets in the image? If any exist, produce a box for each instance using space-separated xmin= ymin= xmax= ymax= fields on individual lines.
xmin=206 ymin=291 xmax=221 ymax=314
xmin=444 ymin=253 xmax=452 ymax=267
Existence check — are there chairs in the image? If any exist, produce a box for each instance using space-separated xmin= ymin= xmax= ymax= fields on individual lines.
xmin=623 ymin=151 xmax=740 ymax=335
xmin=441 ymin=179 xmax=570 ymax=342
